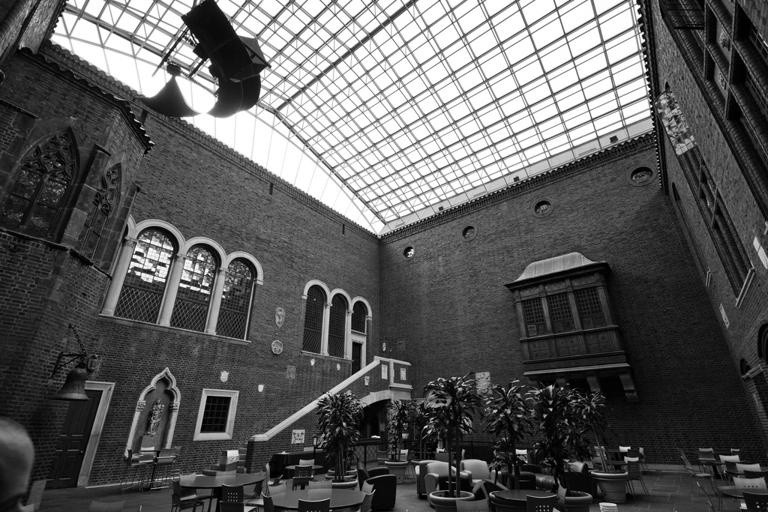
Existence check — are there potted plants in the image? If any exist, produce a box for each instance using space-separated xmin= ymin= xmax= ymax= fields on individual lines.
xmin=315 ymin=390 xmax=368 ymax=489
xmin=384 ymin=396 xmax=412 ymax=484
xmin=408 ymin=369 xmax=488 ymax=510
xmin=525 ymin=379 xmax=610 ymax=512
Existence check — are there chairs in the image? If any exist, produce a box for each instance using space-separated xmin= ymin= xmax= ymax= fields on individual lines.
xmin=524 ymin=492 xmax=557 ymax=512
xmin=121 ymin=444 xmax=184 ymax=493
xmin=493 ymin=443 xmax=651 ymax=502
xmin=171 ymin=459 xmax=395 ymax=511
xmin=677 ymin=444 xmax=768 ymax=511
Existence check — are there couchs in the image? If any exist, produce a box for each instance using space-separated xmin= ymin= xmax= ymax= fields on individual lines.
xmin=410 ymin=458 xmax=497 ymax=500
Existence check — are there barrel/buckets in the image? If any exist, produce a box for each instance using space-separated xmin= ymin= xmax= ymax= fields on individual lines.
xmin=598 ymin=502 xmax=619 ymax=512
